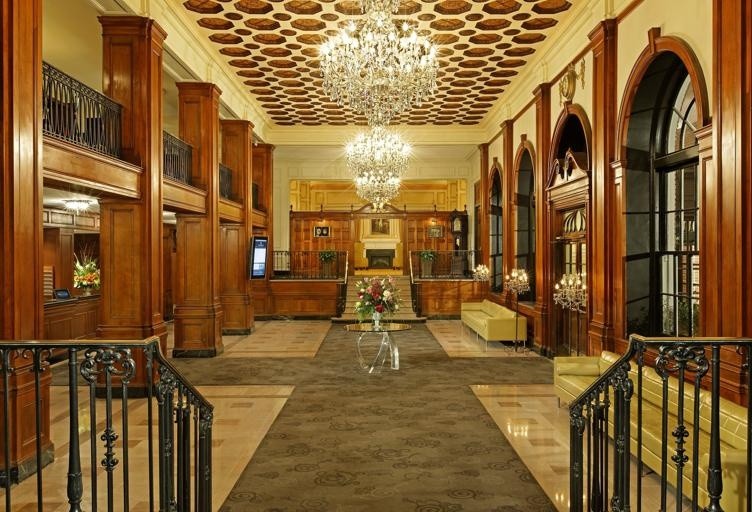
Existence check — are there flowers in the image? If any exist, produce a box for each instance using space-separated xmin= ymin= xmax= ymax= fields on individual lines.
xmin=352 ymin=273 xmax=404 ymax=327
xmin=74 ymin=243 xmax=101 ymax=290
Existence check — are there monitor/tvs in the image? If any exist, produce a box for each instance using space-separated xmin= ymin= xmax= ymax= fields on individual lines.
xmin=249 ymin=235 xmax=268 ymax=279
xmin=52 ymin=288 xmax=73 ymax=299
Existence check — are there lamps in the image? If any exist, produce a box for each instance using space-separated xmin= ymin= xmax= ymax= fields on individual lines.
xmin=316 ymin=0 xmax=440 ymax=126
xmin=504 ymin=268 xmax=530 ymax=352
xmin=343 ymin=127 xmax=414 ymax=207
xmin=474 ymin=264 xmax=491 ymax=302
xmin=553 ymin=274 xmax=587 ymax=356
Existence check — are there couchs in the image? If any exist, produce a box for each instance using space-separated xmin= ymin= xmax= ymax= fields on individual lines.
xmin=553 ymin=350 xmax=748 ymax=512
xmin=461 ymin=299 xmax=527 ymax=353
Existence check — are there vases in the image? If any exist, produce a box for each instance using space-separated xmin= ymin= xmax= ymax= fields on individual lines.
xmin=82 ymin=287 xmax=93 ymax=296
xmin=372 ymin=311 xmax=381 ymax=329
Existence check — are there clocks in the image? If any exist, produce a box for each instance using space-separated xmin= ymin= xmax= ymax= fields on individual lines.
xmin=555 ymin=58 xmax=587 ymax=105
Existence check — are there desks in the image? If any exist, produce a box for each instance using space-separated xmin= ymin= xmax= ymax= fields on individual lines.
xmin=343 ymin=322 xmax=412 ymax=377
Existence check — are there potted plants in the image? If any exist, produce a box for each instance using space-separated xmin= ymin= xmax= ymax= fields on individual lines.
xmin=319 ymin=249 xmax=336 ymax=279
xmin=419 ymin=249 xmax=439 ymax=279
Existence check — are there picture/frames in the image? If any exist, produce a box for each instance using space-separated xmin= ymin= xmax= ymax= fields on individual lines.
xmin=313 ymin=226 xmax=330 ymax=238
xmin=427 ymin=224 xmax=443 ymax=238
